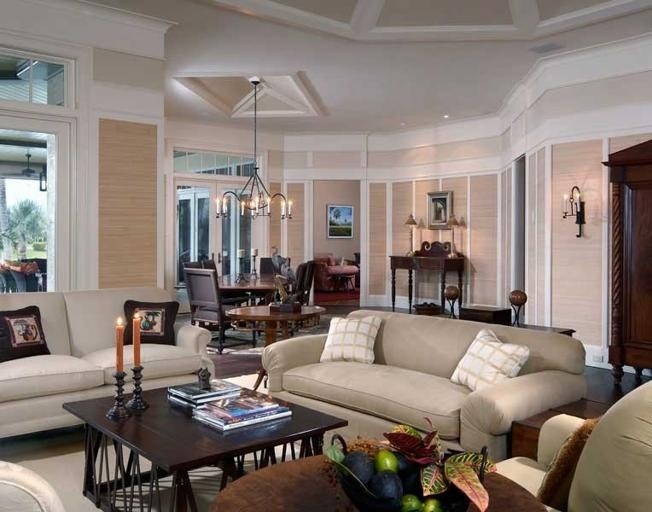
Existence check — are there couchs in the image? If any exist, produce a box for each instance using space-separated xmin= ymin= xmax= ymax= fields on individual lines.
xmin=0 ymin=285 xmax=217 ymax=441
xmin=260 ymin=309 xmax=588 ymax=457
xmin=314 ymin=252 xmax=356 ymax=292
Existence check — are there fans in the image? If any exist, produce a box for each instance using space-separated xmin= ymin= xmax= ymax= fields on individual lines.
xmin=0 ymin=153 xmax=47 ymax=179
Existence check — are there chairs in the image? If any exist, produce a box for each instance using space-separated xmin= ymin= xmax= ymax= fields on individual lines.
xmin=181 ymin=256 xmax=315 ymax=356
xmin=0 ymin=460 xmax=65 ymax=512
xmin=0 ymin=258 xmax=47 ymax=292
xmin=490 ymin=379 xmax=652 ymax=512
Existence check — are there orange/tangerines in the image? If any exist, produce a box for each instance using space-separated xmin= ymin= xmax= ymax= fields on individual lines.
xmin=376 ymin=451 xmax=399 ymax=473
xmin=401 ymin=495 xmax=441 ymax=512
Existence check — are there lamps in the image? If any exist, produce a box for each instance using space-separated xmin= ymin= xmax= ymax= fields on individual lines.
xmin=446 ymin=214 xmax=459 ymax=259
xmin=561 ymin=185 xmax=586 ymax=237
xmin=405 ymin=214 xmax=418 ymax=257
xmin=215 ymin=80 xmax=293 ymax=220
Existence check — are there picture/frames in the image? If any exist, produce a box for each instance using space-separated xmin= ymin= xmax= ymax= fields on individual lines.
xmin=426 ymin=190 xmax=453 ymax=230
xmin=326 ymin=204 xmax=354 ymax=239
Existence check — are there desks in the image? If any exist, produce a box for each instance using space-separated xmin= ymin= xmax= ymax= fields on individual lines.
xmin=388 ymin=240 xmax=466 ymax=314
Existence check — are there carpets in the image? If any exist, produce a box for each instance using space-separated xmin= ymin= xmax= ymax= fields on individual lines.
xmin=14 ymin=370 xmax=315 ymax=512
xmin=314 ymin=287 xmax=360 ymax=304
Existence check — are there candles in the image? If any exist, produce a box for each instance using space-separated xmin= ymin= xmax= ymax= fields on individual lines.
xmin=114 ymin=315 xmax=127 ymax=372
xmin=132 ymin=311 xmax=142 ymax=367
xmin=237 ymin=248 xmax=245 ymax=258
xmin=251 ymin=248 xmax=258 ymax=257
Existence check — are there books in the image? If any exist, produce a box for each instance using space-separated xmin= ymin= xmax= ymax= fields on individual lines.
xmin=166 ymin=379 xmax=293 ymax=432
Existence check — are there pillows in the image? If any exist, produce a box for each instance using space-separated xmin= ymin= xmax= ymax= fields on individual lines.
xmin=448 ymin=327 xmax=530 ymax=393
xmin=536 ymin=415 xmax=601 ymax=512
xmin=120 ymin=300 xmax=180 ymax=346
xmin=325 ymin=264 xmax=360 ymax=274
xmin=0 ymin=305 xmax=50 ymax=363
xmin=319 ymin=316 xmax=383 ymax=365
